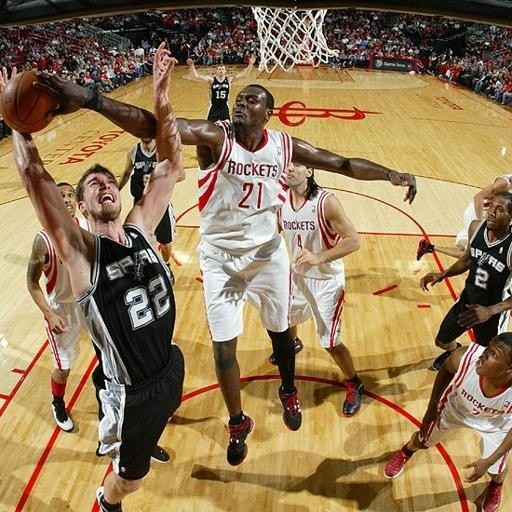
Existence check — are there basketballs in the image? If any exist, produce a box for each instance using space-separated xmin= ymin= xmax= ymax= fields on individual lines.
xmin=1 ymin=71 xmax=59 ymax=132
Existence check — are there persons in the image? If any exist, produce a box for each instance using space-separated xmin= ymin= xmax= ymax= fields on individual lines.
xmin=1 ymin=9 xmax=512 ymax=123
xmin=33 ymin=69 xmax=417 ymax=466
xmin=118 ymin=139 xmax=186 ymax=285
xmin=416 ymin=174 xmax=512 ymax=261
xmin=270 ymin=161 xmax=364 ymax=416
xmin=384 ymin=332 xmax=512 ymax=512
xmin=0 ymin=42 xmax=184 ymax=512
xmin=420 ymin=192 xmax=512 ymax=372
xmin=27 ymin=183 xmax=92 ymax=432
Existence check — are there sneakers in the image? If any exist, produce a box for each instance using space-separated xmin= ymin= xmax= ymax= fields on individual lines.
xmin=269 ymin=337 xmax=303 ymax=365
xmin=417 ymin=240 xmax=430 ymax=261
xmin=432 ymin=342 xmax=462 ymax=371
xmin=343 ymin=372 xmax=364 ymax=416
xmin=384 ymin=443 xmax=417 ymax=479
xmin=227 ymin=414 xmax=255 ymax=466
xmin=52 ymin=400 xmax=74 ymax=431
xmin=278 ymin=384 xmax=302 ymax=430
xmin=150 ymin=445 xmax=170 ymax=463
xmin=166 ymin=262 xmax=174 ymax=285
xmin=96 ymin=487 xmax=121 ymax=512
xmin=481 ymin=478 xmax=502 ymax=512
